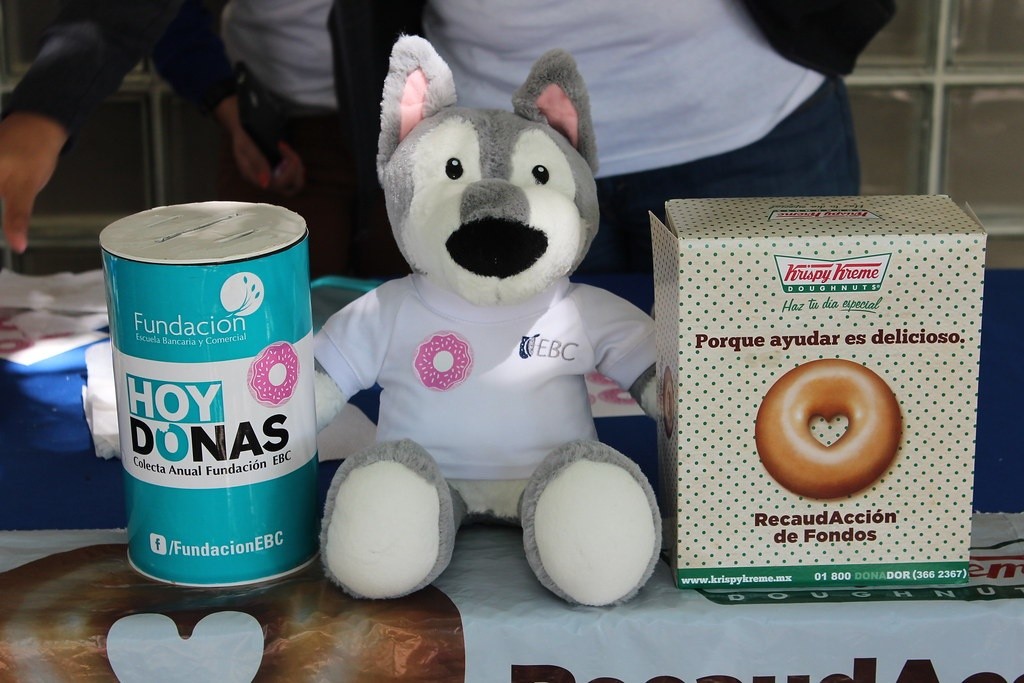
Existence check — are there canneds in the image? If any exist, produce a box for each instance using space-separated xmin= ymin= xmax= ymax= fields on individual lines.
xmin=98 ymin=200 xmax=323 ymax=589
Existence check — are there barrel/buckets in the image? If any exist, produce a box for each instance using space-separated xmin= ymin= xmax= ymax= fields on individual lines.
xmin=97 ymin=201 xmax=321 ymax=592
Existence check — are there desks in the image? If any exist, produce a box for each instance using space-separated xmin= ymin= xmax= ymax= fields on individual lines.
xmin=0 ymin=267 xmax=1024 ymax=683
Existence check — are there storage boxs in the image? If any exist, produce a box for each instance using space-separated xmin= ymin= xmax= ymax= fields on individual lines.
xmin=647 ymin=196 xmax=990 ymax=592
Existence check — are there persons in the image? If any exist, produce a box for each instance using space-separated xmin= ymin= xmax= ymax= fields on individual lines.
xmin=2 ymin=2 xmax=447 ymax=288
xmin=323 ymin=0 xmax=897 ymax=353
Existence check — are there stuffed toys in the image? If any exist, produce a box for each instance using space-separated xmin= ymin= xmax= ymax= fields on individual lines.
xmin=315 ymin=34 xmax=664 ymax=609
